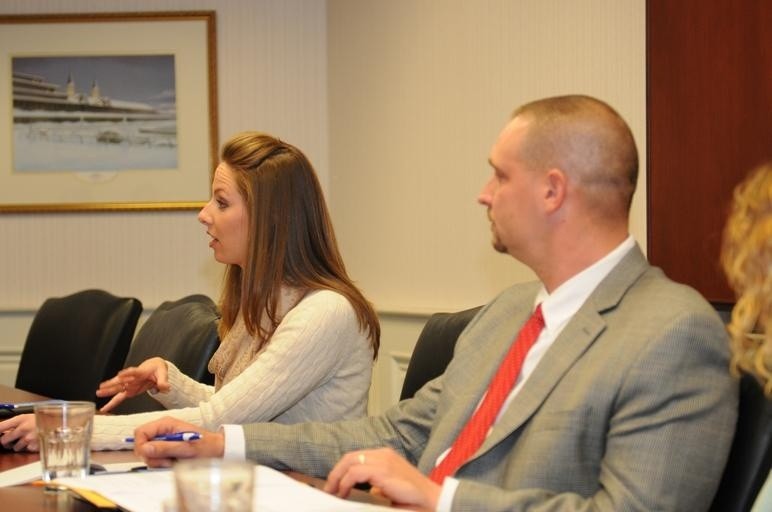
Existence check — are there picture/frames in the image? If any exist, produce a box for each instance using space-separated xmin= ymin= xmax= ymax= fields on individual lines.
xmin=1 ymin=8 xmax=222 ymax=216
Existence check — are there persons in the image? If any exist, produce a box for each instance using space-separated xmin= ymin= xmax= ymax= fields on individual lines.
xmin=721 ymin=162 xmax=772 ymax=511
xmin=132 ymin=94 xmax=739 ymax=511
xmin=1 ymin=130 xmax=382 ymax=455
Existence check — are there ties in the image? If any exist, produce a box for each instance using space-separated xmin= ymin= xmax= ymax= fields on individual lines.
xmin=393 ymin=300 xmax=547 ymax=507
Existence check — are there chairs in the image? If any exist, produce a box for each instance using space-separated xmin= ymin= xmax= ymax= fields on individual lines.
xmin=708 ymin=302 xmax=772 ymax=512
xmin=398 ymin=304 xmax=486 ymax=403
xmin=14 ymin=287 xmax=143 ymax=410
xmin=112 ymin=292 xmax=225 ymax=418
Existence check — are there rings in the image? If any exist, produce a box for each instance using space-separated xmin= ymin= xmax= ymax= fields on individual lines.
xmin=356 ymin=453 xmax=365 ymax=463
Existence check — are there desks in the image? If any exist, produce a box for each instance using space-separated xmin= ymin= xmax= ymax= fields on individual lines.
xmin=1 ymin=379 xmax=432 ymax=511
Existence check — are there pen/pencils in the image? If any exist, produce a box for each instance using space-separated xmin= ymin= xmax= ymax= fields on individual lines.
xmin=123 ymin=431 xmax=202 ymax=441
xmin=0 ymin=404 xmax=19 ymax=409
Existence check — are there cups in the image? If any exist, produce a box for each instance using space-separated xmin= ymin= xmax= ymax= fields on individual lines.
xmin=34 ymin=401 xmax=95 ymax=493
xmin=174 ymin=458 xmax=259 ymax=512
